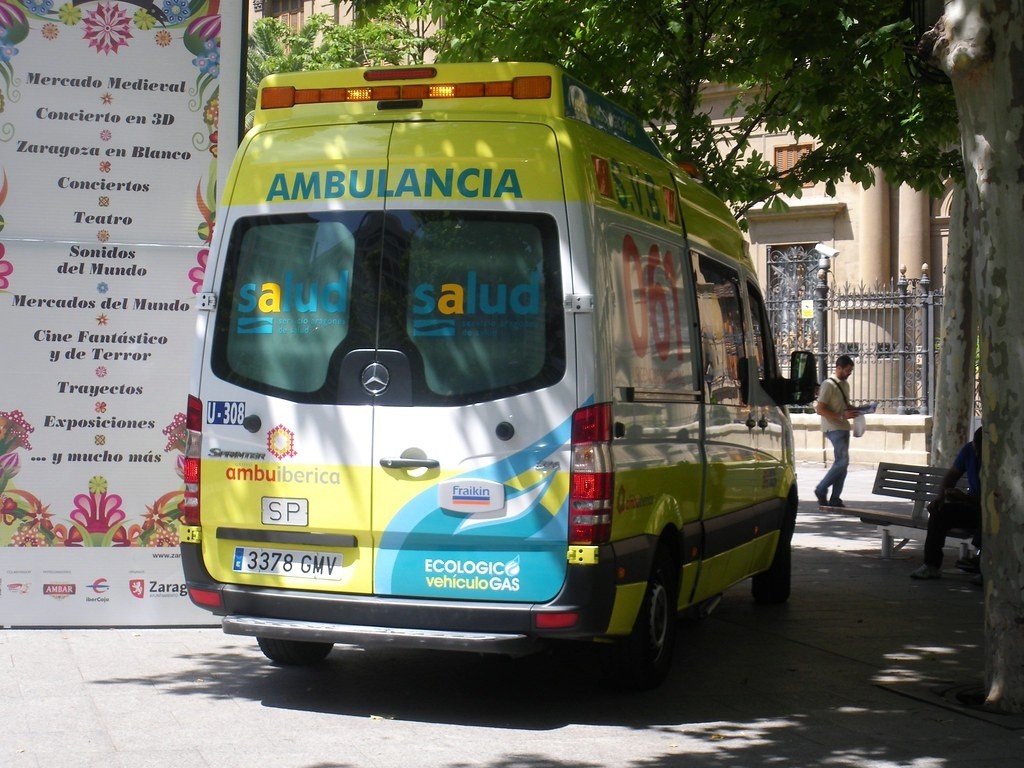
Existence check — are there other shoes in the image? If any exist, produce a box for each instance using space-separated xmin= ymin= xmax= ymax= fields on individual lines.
xmin=815 ymin=489 xmax=827 ymax=505
xmin=829 ymin=503 xmax=845 ymax=507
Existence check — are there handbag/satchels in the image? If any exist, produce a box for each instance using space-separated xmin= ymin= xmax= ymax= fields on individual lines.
xmin=852 ymin=414 xmax=867 ymax=438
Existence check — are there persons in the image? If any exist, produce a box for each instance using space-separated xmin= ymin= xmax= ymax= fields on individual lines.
xmin=909 ymin=426 xmax=982 ymax=579
xmin=814 ymin=355 xmax=870 ymax=506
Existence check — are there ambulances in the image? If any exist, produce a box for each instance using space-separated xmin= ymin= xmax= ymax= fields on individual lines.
xmin=175 ymin=60 xmax=820 ymax=696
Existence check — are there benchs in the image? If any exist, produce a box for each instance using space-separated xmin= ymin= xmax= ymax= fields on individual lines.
xmin=820 ymin=462 xmax=978 ymax=576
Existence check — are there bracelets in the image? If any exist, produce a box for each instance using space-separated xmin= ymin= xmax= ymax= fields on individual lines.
xmin=840 ymin=413 xmax=844 ymax=422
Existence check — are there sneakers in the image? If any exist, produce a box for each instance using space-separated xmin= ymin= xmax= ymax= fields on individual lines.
xmin=954 ymin=558 xmax=979 ymax=573
xmin=910 ymin=565 xmax=942 ymax=578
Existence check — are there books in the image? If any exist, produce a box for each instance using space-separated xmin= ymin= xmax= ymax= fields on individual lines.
xmin=856 ymin=403 xmax=877 ymax=414
